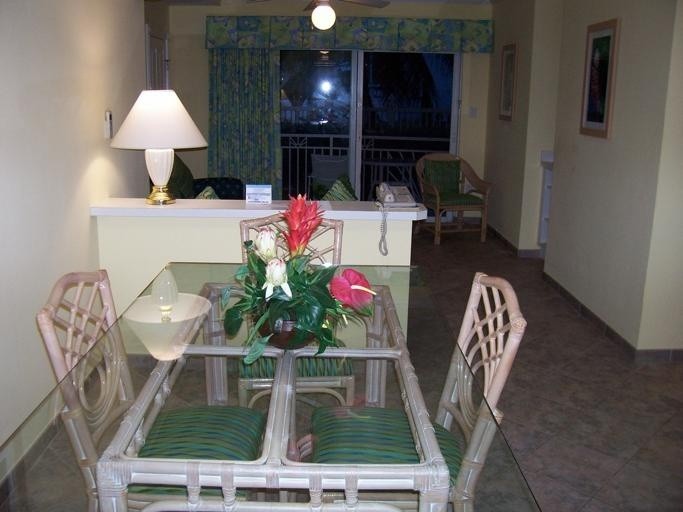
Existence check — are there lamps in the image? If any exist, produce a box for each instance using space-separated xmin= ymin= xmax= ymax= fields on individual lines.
xmin=106 ymin=87 xmax=210 ymax=206
xmin=303 ymin=2 xmax=340 ymax=31
xmin=121 ymin=266 xmax=215 ymax=362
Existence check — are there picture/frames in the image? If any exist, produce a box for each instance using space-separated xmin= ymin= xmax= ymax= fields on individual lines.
xmin=575 ymin=13 xmax=622 ymax=140
xmin=494 ymin=39 xmax=520 ymax=126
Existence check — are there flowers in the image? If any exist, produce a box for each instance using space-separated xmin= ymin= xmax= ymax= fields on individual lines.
xmin=216 ymin=191 xmax=378 ymax=365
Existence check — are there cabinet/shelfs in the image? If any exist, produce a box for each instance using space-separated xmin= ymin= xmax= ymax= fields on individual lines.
xmin=538 ymin=150 xmax=555 ymax=245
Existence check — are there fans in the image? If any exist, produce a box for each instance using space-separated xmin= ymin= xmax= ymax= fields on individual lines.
xmin=247 ymin=0 xmax=391 ymax=12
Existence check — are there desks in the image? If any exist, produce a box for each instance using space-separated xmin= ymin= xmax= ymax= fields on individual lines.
xmin=0 ymin=258 xmax=550 ymax=512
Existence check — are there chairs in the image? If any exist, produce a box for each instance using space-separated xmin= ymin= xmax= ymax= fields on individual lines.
xmin=32 ymin=266 xmax=269 ymax=512
xmin=236 ymin=212 xmax=356 ymax=410
xmin=412 ymin=150 xmax=496 ymax=248
xmin=308 ymin=267 xmax=530 ymax=512
xmin=144 ymin=154 xmax=245 ymax=199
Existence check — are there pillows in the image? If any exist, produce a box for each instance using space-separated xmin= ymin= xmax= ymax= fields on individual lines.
xmin=193 ymin=185 xmax=220 ymax=200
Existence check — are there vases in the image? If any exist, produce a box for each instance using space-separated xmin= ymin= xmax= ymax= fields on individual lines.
xmin=251 ymin=307 xmax=318 ymax=349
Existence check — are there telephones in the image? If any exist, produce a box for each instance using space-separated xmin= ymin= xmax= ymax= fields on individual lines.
xmin=376 ymin=182 xmax=417 ymax=207
